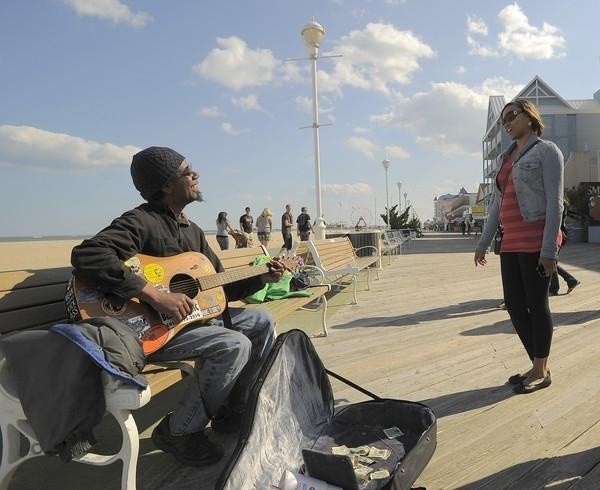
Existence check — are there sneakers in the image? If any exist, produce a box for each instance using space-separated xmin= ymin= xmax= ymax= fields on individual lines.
xmin=566 ymin=279 xmax=581 ymax=295
xmin=548 ymin=291 xmax=559 ymax=297
xmin=496 ymin=300 xmax=507 ymax=310
xmin=210 ymin=412 xmax=241 ymax=436
xmin=151 ymin=412 xmax=225 ymax=467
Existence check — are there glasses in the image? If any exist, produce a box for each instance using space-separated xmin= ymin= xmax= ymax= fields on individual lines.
xmin=502 ymin=108 xmax=526 ymax=126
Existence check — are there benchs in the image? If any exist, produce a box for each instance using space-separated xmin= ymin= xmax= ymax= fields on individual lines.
xmin=286 ymin=240 xmax=310 ymax=260
xmin=299 ymin=235 xmax=383 ymax=307
xmin=377 ymin=229 xmax=419 ymax=265
xmin=0 ymin=233 xmax=335 ymax=490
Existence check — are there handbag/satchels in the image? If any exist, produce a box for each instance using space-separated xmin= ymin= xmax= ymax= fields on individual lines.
xmin=494 ymin=227 xmax=504 ymax=255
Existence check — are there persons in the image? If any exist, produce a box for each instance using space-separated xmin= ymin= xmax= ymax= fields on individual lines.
xmin=497 ymin=301 xmax=507 ymax=310
xmin=472 ymin=98 xmax=564 ymax=393
xmin=295 ymin=206 xmax=315 ymax=241
xmin=216 ymin=211 xmax=236 ymax=251
xmin=239 ymin=207 xmax=253 ymax=242
xmin=67 ymin=145 xmax=285 ymax=468
xmin=560 ymin=191 xmax=582 ymax=294
xmin=467 ymin=213 xmax=473 ymax=236
xmin=314 ymin=213 xmax=327 ymax=226
xmin=549 ymin=228 xmax=562 ymax=296
xmin=461 ymin=218 xmax=467 ymax=235
xmin=256 ymin=207 xmax=273 ymax=248
xmin=281 ymin=204 xmax=294 ymax=250
xmin=413 ymin=225 xmax=424 ymax=237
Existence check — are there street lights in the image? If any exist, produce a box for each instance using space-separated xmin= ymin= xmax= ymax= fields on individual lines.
xmin=298 ymin=15 xmax=329 ymax=240
xmin=381 ymin=157 xmax=393 ymax=231
xmin=395 ymin=180 xmax=421 ymax=227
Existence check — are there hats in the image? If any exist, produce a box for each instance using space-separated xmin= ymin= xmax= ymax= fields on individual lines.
xmin=130 ymin=146 xmax=186 ymax=200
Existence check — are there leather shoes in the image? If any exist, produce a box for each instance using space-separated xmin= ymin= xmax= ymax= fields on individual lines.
xmin=508 ymin=367 xmax=551 ymax=384
xmin=512 ymin=373 xmax=551 ymax=394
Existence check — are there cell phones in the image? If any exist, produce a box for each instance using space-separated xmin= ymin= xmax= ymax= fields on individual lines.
xmin=535 ymin=263 xmax=546 ymax=280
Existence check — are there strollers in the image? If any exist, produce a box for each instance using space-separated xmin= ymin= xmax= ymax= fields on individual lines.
xmin=228 ymin=227 xmax=256 ymax=248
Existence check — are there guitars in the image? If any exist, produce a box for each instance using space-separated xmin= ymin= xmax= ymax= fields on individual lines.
xmin=64 ymin=249 xmax=305 ymax=358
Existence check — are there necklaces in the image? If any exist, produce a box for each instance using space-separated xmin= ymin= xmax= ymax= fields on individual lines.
xmin=511 ymin=161 xmax=514 ymax=166
